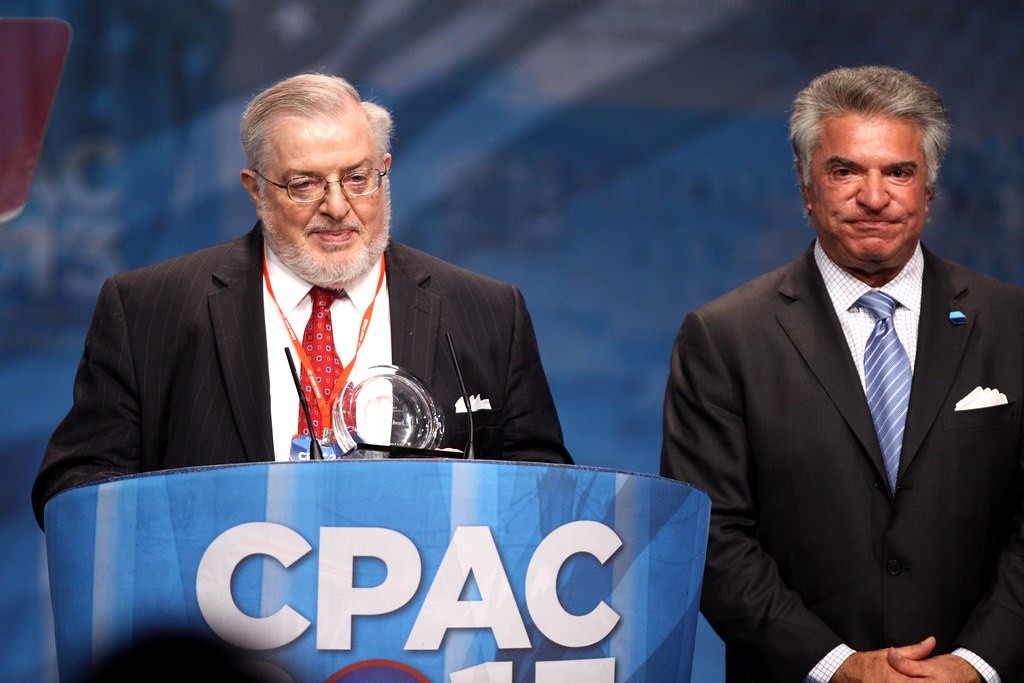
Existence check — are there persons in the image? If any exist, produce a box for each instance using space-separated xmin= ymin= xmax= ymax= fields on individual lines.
xmin=32 ymin=73 xmax=576 ymax=536
xmin=659 ymin=64 xmax=1024 ymax=683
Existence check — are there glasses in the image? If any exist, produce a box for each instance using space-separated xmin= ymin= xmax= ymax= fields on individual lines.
xmin=255 ymin=159 xmax=388 ymax=204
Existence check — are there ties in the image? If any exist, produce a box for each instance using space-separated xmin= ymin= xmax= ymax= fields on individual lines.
xmin=298 ymin=285 xmax=357 ymax=443
xmin=854 ymin=290 xmax=913 ymax=496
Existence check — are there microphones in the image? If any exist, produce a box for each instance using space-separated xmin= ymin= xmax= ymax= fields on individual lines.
xmin=446 ymin=333 xmax=475 ymax=459
xmin=285 ymin=347 xmax=325 ymax=460
